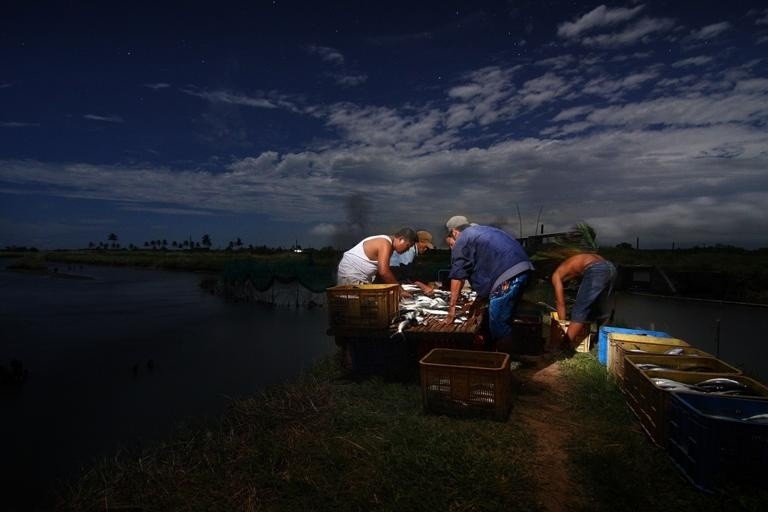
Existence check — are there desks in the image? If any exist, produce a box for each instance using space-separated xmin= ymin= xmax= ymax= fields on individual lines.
xmin=325 ymin=291 xmax=485 ymax=376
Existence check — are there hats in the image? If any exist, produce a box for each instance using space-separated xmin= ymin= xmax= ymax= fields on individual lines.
xmin=416 ymin=231 xmax=434 ymax=250
xmin=446 ymin=215 xmax=470 ymax=237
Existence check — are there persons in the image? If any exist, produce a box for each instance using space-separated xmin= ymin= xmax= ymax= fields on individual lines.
xmin=545 ymin=252 xmax=618 ymax=358
xmin=439 ymin=215 xmax=536 ymax=371
xmin=374 ymin=230 xmax=434 ymax=297
xmin=335 ymin=227 xmax=419 ymax=373
xmin=446 ymin=231 xmax=489 ymax=314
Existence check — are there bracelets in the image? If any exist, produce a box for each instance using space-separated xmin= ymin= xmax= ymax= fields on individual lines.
xmin=449 ymin=301 xmax=455 ymax=307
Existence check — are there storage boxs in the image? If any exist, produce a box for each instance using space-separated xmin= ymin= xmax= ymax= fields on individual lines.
xmin=326 ymin=282 xmax=401 ymax=333
xmin=418 ymin=347 xmax=514 ymax=423
xmin=597 ymin=325 xmax=768 ymax=495
xmin=550 ymin=318 xmax=591 ymax=354
